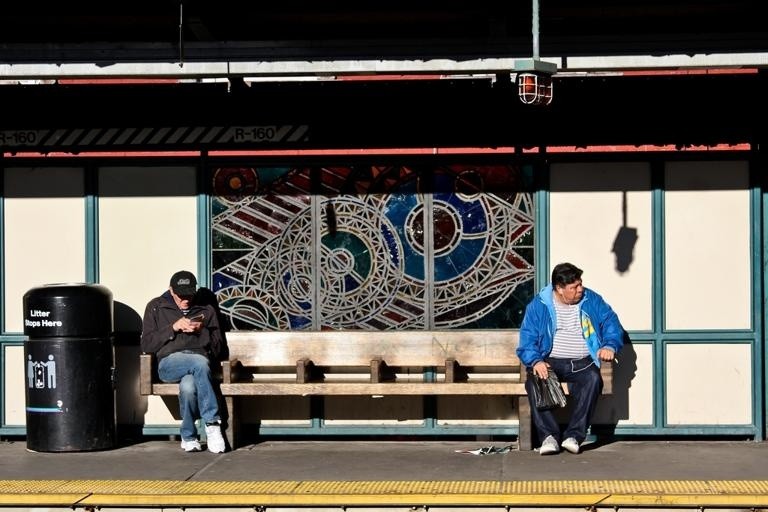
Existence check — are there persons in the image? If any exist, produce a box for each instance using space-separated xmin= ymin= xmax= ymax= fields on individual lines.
xmin=515 ymin=263 xmax=624 ymax=456
xmin=140 ymin=271 xmax=227 ymax=454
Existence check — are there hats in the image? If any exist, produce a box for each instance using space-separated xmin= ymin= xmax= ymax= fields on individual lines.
xmin=170 ymin=269 xmax=198 ymax=298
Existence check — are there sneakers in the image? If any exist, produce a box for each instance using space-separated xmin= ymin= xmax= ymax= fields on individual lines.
xmin=538 ymin=435 xmax=560 ymax=456
xmin=204 ymin=420 xmax=226 ymax=453
xmin=562 ymin=436 xmax=581 ymax=453
xmin=181 ymin=436 xmax=202 ymax=453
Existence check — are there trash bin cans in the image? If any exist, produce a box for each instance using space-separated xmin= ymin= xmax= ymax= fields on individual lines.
xmin=22 ymin=283 xmax=119 ymax=454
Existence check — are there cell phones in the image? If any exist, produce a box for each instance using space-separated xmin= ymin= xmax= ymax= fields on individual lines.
xmin=189 ymin=315 xmax=203 ymax=323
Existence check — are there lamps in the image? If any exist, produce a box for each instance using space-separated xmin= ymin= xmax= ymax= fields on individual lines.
xmin=511 ymin=0 xmax=558 ymax=106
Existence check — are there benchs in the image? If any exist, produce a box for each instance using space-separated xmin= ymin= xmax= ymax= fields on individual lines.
xmin=140 ymin=348 xmax=613 ymax=452
